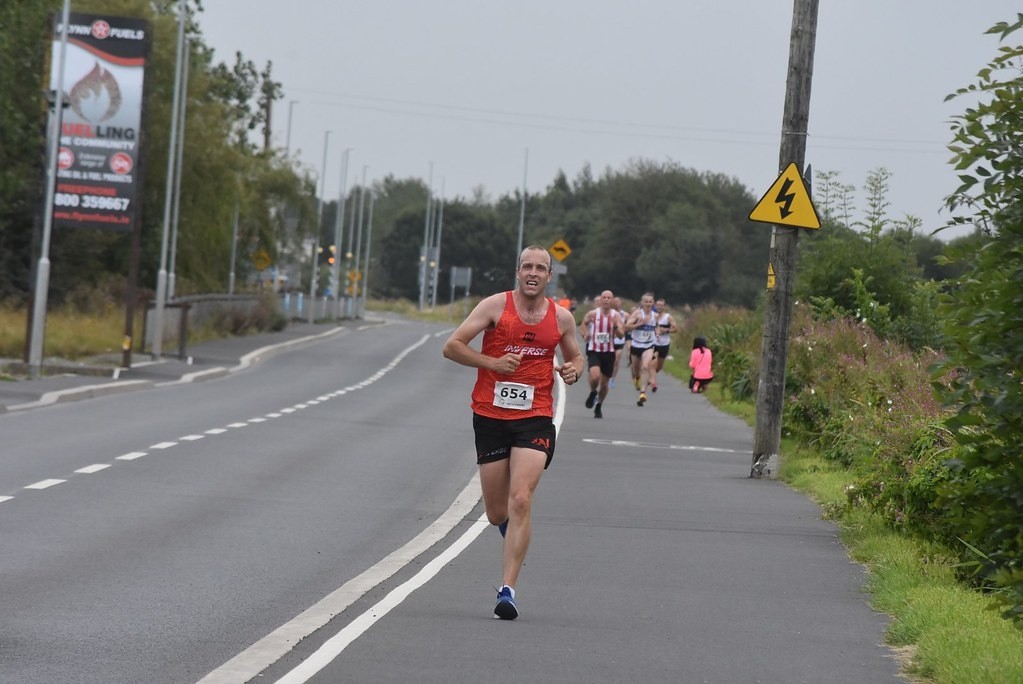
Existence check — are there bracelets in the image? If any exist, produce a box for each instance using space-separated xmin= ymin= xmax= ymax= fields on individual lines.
xmin=576 ymin=372 xmax=578 ymax=382
xmin=666 ymin=328 xmax=669 ymax=333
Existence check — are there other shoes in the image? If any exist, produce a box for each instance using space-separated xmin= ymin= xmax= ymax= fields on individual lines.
xmin=585 ymin=391 xmax=598 ymax=409
xmin=692 ymin=381 xmax=700 ymax=393
xmin=702 ymin=384 xmax=707 ymax=392
xmin=637 ymin=393 xmax=647 ymax=406
xmin=651 ymin=384 xmax=657 ymax=392
xmin=609 ymin=378 xmax=615 ymax=388
xmin=633 ymin=375 xmax=640 ymax=391
xmin=594 ymin=403 xmax=602 ymax=418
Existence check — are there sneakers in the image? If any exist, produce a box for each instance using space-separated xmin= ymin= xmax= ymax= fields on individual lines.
xmin=499 ymin=518 xmax=509 ymax=539
xmin=492 ymin=581 xmax=518 ymax=620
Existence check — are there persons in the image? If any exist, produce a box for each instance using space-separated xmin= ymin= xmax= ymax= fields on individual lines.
xmin=444 ymin=245 xmax=584 ymax=619
xmin=581 ymin=290 xmax=623 ymax=418
xmin=571 ymin=296 xmax=578 ymax=316
xmin=688 ymin=336 xmax=714 ymax=392
xmin=625 ymin=292 xmax=660 ymax=407
xmin=586 ymin=296 xmax=678 ymax=393
xmin=558 ymin=294 xmax=570 ymax=311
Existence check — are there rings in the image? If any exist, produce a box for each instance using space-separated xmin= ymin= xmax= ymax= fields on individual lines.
xmin=567 ymin=375 xmax=569 ymax=379
